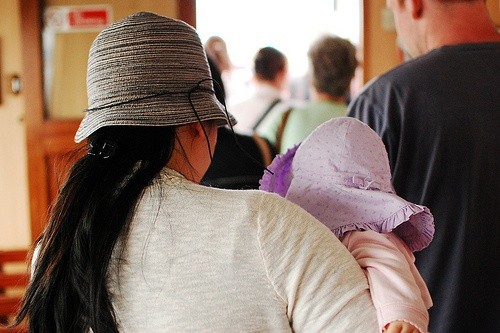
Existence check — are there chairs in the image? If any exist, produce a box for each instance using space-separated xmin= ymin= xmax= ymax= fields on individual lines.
xmin=0 ymin=248 xmax=33 ymax=333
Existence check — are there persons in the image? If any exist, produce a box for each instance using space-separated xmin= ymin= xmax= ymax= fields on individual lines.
xmin=224 ymin=46 xmax=292 ymax=138
xmin=260 ymin=34 xmax=360 ymax=158
xmin=201 ymin=35 xmax=256 ymax=112
xmin=259 ymin=117 xmax=435 ymax=333
xmin=9 ymin=11 xmax=383 ymax=333
xmin=345 ymin=0 xmax=500 ymax=333
xmin=205 ymin=57 xmax=284 ymax=192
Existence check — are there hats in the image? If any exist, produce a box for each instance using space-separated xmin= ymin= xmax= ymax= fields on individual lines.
xmin=74 ymin=11 xmax=238 ymax=144
xmin=258 ymin=117 xmax=434 ymax=252
